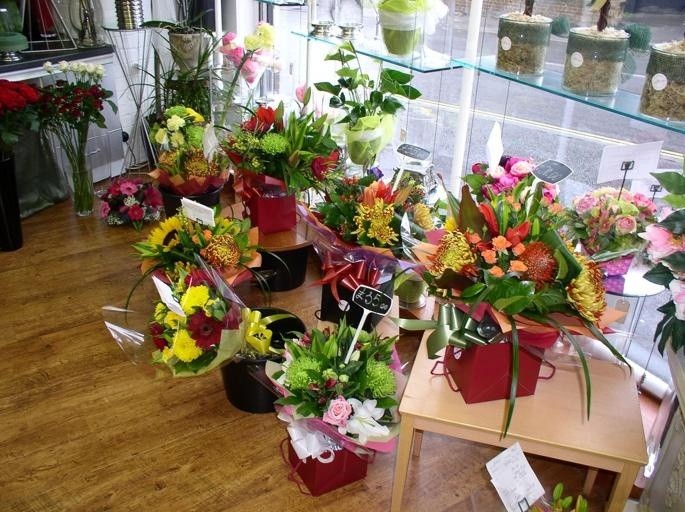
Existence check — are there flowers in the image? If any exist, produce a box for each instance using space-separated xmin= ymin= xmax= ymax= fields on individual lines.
xmin=0 ymin=77 xmax=44 ymax=151
xmin=265 ymin=81 xmax=685 ymax=497
xmin=29 ymin=61 xmax=118 ymax=213
xmin=123 ymin=202 xmax=295 ymax=378
xmin=96 ymin=176 xmax=164 ymax=232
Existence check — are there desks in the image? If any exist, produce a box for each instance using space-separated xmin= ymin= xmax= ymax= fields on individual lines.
xmin=101 ymin=21 xmax=157 ymax=177
xmin=390 ymin=328 xmax=649 ymax=512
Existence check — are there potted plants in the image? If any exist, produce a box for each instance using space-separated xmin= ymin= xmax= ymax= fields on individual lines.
xmin=139 ymin=1 xmax=219 ymax=74
xmin=495 ymin=1 xmax=553 ymax=77
xmin=561 ymin=1 xmax=631 ymax=97
xmin=313 ymin=38 xmax=422 ymax=165
xmin=639 ymin=32 xmax=685 ymax=122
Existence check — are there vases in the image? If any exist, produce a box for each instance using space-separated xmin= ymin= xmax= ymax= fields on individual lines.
xmin=67 ymin=166 xmax=94 ymax=217
xmin=0 ymin=151 xmax=24 ymax=253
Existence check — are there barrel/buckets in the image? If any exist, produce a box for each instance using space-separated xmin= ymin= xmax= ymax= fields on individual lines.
xmin=220 ymin=307 xmax=305 ymax=414
xmin=316 ymin=274 xmax=371 ymax=335
xmin=157 ymin=183 xmax=221 ymax=221
